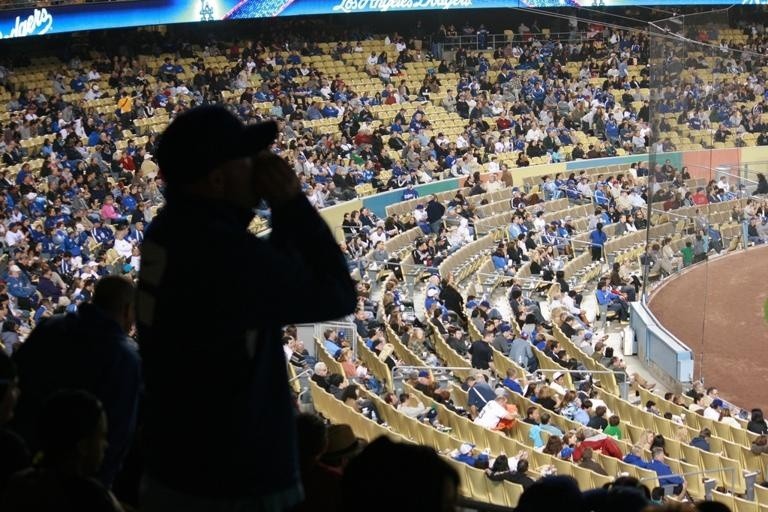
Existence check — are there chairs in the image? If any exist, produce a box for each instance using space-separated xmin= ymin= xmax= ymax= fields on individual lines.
xmin=0 ymin=28 xmax=768 ymax=512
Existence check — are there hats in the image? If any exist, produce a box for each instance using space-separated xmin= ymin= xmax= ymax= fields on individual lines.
xmin=323 ymin=424 xmax=364 ymax=453
xmin=339 ymin=272 xmax=615 ymax=458
xmin=159 ymin=104 xmax=277 ymax=184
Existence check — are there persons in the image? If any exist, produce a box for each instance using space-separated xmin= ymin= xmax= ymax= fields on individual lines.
xmin=0 ymin=9 xmax=768 ymax=512
